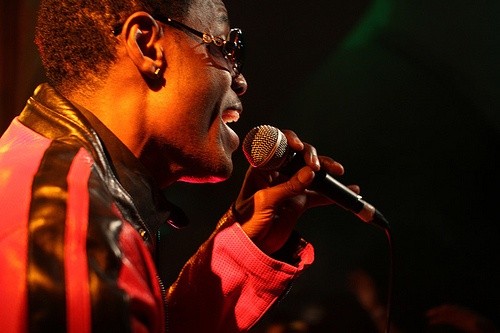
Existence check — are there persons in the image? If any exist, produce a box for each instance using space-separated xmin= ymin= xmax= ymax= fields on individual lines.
xmin=267 ymin=269 xmax=500 ymax=333
xmin=0 ymin=0 xmax=360 ymax=333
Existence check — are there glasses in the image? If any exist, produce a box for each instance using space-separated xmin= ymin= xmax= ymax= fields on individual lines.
xmin=112 ymin=12 xmax=242 ymax=77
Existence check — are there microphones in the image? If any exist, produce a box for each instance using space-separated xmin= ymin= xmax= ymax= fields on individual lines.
xmin=242 ymin=124 xmax=390 ymax=232
